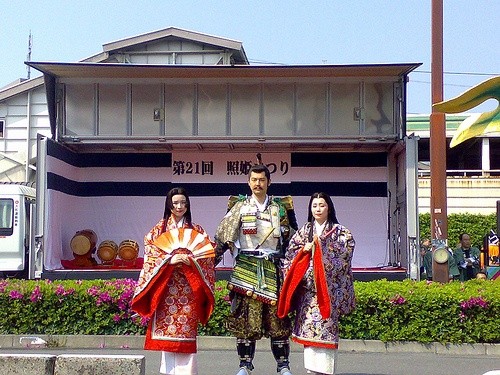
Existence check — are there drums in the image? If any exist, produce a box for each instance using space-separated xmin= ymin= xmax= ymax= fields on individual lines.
xmin=97 ymin=240 xmax=118 ymax=262
xmin=117 ymin=240 xmax=139 ymax=261
xmin=70 ymin=229 xmax=98 ymax=256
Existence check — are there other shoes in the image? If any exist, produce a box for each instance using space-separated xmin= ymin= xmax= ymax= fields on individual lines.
xmin=307 ymin=371 xmax=319 ymax=375
xmin=278 ymin=368 xmax=292 ymax=375
xmin=236 ymin=366 xmax=255 ymax=375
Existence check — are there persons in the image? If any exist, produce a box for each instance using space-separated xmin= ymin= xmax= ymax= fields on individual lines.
xmin=131 ymin=187 xmax=217 ymax=375
xmin=419 ymin=233 xmax=487 ymax=280
xmin=211 ymin=163 xmax=299 ymax=375
xmin=276 ymin=192 xmax=355 ymax=375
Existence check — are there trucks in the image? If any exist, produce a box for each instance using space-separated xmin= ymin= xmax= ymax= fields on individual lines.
xmin=0 ymin=62 xmax=424 ymax=282
